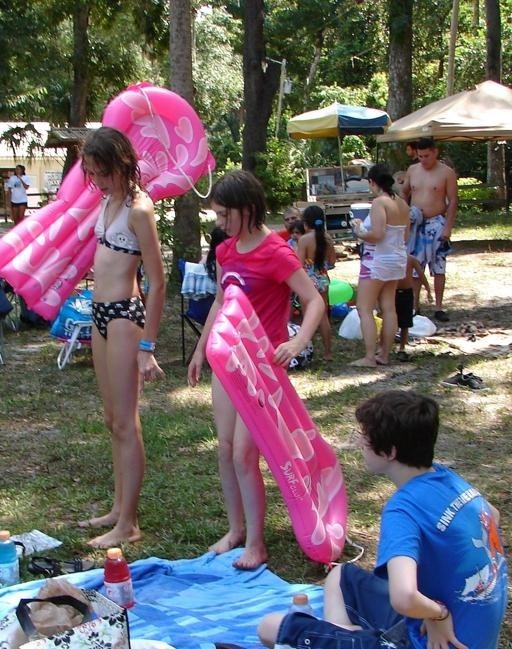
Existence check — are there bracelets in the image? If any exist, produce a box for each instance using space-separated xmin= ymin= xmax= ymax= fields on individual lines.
xmin=139 ymin=338 xmax=155 ymax=352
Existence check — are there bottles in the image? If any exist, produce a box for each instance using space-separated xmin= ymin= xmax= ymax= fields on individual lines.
xmin=0 ymin=530 xmax=20 ymax=584
xmin=287 ymin=594 xmax=313 ymax=617
xmin=104 ymin=547 xmax=135 ymax=610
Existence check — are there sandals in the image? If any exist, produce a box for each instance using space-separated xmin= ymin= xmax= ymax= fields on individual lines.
xmin=27 ymin=555 xmax=93 ymax=577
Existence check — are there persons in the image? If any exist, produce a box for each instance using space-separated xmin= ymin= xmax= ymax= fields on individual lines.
xmin=405 ymin=140 xmax=420 ymax=163
xmin=4 ymin=164 xmax=29 ymax=224
xmin=186 ymin=168 xmax=326 ymax=572
xmin=206 ymin=205 xmax=336 ymax=363
xmin=255 ymin=389 xmax=508 ymax=649
xmin=76 ymin=127 xmax=167 ymax=550
xmin=350 ymin=163 xmax=413 ymax=368
xmin=379 ymin=256 xmax=433 ymax=362
xmin=401 ymin=136 xmax=459 ymax=323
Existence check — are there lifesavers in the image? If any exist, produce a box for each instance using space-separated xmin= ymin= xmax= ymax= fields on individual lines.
xmin=329 ymin=280 xmax=353 ymax=305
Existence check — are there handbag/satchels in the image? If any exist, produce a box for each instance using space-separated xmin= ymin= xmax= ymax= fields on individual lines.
xmin=50 ymin=296 xmax=92 ymax=339
xmin=0 ymin=589 xmax=130 ymax=649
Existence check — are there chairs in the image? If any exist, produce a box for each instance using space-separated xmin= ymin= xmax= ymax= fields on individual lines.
xmin=50 ymin=272 xmax=148 ymax=373
xmin=177 ymin=257 xmax=217 ymax=369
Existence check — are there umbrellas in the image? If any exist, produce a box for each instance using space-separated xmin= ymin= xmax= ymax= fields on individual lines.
xmin=287 ymin=100 xmax=393 ymax=190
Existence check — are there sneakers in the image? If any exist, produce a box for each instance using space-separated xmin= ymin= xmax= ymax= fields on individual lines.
xmin=398 ymin=351 xmax=408 ymax=361
xmin=434 ymin=311 xmax=449 ymax=321
xmin=442 ymin=372 xmax=491 ymax=392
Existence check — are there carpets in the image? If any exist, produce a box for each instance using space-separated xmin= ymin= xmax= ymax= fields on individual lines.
xmin=0 ymin=541 xmax=333 ymax=649
